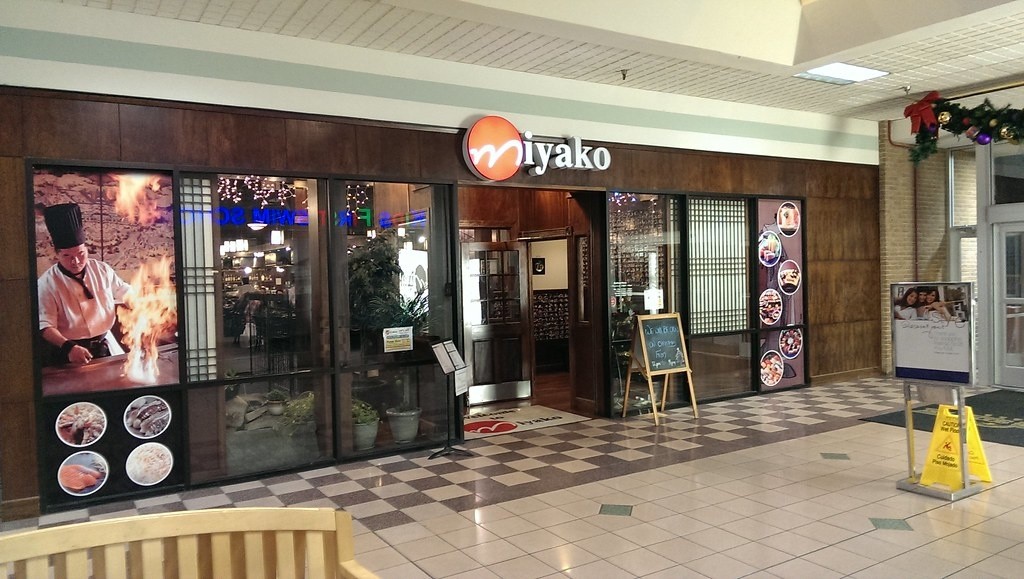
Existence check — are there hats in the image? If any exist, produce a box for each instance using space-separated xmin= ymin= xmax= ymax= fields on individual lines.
xmin=42 ymin=203 xmax=85 ymax=249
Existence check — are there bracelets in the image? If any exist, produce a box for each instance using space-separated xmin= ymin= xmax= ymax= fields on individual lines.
xmin=61 ymin=339 xmax=77 ymax=355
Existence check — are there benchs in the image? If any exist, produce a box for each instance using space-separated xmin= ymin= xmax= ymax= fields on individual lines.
xmin=0 ymin=506 xmax=381 ymax=578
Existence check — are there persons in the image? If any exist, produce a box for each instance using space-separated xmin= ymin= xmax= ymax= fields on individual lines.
xmin=913 ymin=286 xmax=945 ymax=318
xmin=922 ymin=287 xmax=953 ymax=320
xmin=38 ymin=201 xmax=142 ymax=367
xmin=894 ymin=288 xmax=920 ymax=321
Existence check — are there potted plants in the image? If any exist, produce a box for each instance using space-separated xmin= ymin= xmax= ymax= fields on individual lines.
xmin=265 ymin=389 xmax=287 ymax=415
xmin=274 ymin=395 xmax=321 ymax=464
xmin=364 ymin=282 xmax=436 ymax=446
xmin=225 ymin=368 xmax=240 ymax=402
xmin=351 ymin=399 xmax=381 ymax=451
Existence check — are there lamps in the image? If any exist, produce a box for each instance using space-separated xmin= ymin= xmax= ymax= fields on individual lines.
xmin=218 ymin=221 xmax=285 ymax=256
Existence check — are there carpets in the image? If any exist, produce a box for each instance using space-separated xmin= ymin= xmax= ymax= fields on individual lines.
xmin=857 ymin=388 xmax=1024 ymax=448
xmin=462 ymin=404 xmax=593 ymax=440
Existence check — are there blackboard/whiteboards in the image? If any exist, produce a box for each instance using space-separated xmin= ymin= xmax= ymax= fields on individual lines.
xmin=641 ymin=317 xmax=687 ymax=371
